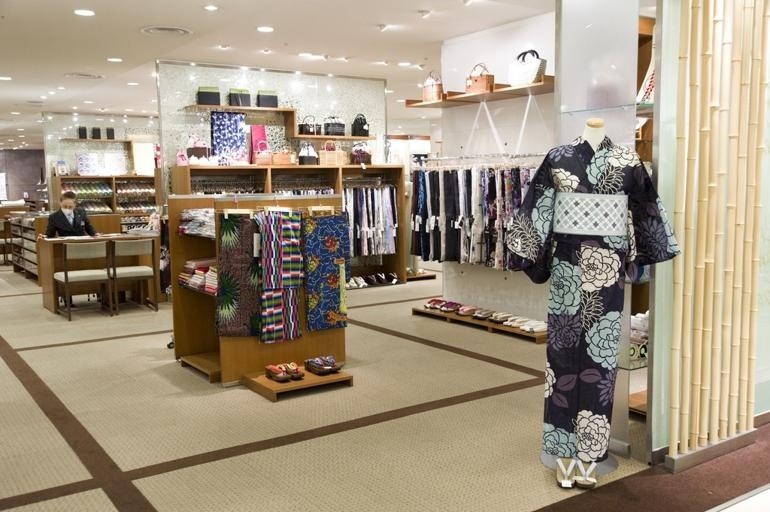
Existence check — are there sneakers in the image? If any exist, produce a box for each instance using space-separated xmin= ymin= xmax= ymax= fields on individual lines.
xmin=346 ymin=271 xmax=401 ymax=289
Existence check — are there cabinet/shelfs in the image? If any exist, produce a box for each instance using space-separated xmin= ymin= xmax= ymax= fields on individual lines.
xmin=166 ymin=192 xmax=354 ymax=398
xmin=52 ymin=176 xmax=157 ymax=233
xmin=0 ymin=200 xmax=121 ymax=288
xmin=168 ymin=162 xmax=409 ymax=287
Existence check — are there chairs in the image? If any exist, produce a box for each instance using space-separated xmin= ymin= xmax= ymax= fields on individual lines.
xmin=109 ymin=237 xmax=160 ymax=314
xmin=56 ymin=239 xmax=113 ymax=321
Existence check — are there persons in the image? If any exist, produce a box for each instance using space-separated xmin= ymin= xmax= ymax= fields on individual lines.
xmin=37 ymin=191 xmax=102 ymax=307
xmin=507 ymin=119 xmax=681 ymax=490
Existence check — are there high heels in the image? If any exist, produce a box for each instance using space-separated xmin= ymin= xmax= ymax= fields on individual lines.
xmin=305 ymin=357 xmax=341 ymax=373
xmin=265 ymin=363 xmax=304 ymax=381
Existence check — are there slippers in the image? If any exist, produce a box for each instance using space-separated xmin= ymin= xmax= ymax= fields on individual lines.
xmin=556 ymin=457 xmax=597 ymax=488
xmin=424 ymin=299 xmax=548 ymax=332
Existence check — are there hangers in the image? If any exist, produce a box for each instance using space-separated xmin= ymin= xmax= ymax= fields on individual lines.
xmin=414 ymin=152 xmax=544 ymax=170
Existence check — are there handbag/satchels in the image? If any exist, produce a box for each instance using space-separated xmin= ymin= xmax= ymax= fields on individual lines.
xmin=422 ymin=70 xmax=441 ymax=101
xmin=466 ymin=63 xmax=494 ymax=93
xmin=508 ymin=51 xmax=546 ymax=87
xmin=299 ymin=114 xmax=369 ymax=136
xmin=254 ymin=140 xmax=371 ymax=165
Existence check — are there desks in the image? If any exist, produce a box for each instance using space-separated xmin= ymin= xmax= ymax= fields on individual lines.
xmin=36 ymin=232 xmax=160 ymax=315
